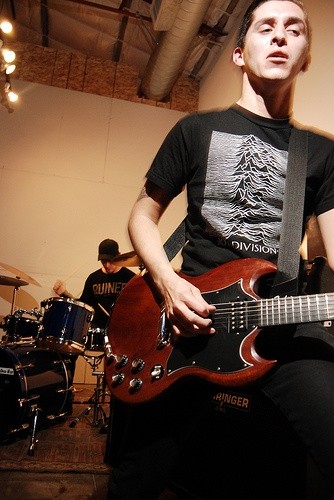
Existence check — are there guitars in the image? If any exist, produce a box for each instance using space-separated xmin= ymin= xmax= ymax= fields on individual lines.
xmin=103 ymin=256 xmax=333 ymax=407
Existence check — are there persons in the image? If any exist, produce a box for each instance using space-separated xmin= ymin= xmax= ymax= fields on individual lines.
xmin=129 ymin=0 xmax=334 ymax=500
xmin=54 ymin=239 xmax=137 ymax=328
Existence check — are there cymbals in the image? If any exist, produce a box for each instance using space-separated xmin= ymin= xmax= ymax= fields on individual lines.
xmin=111 ymin=249 xmax=145 ymax=266
xmin=0 ymin=261 xmax=42 ymax=287
xmin=0 ymin=275 xmax=29 ymax=287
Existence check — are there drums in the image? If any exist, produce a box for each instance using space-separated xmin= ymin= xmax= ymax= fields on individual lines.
xmin=3 ymin=315 xmax=42 ymax=342
xmin=85 ymin=329 xmax=105 ymax=352
xmin=37 ymin=296 xmax=95 ymax=355
xmin=0 ymin=342 xmax=74 ymax=437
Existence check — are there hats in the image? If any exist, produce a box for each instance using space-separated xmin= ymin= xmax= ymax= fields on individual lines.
xmin=98 ymin=239 xmax=119 ymax=261
